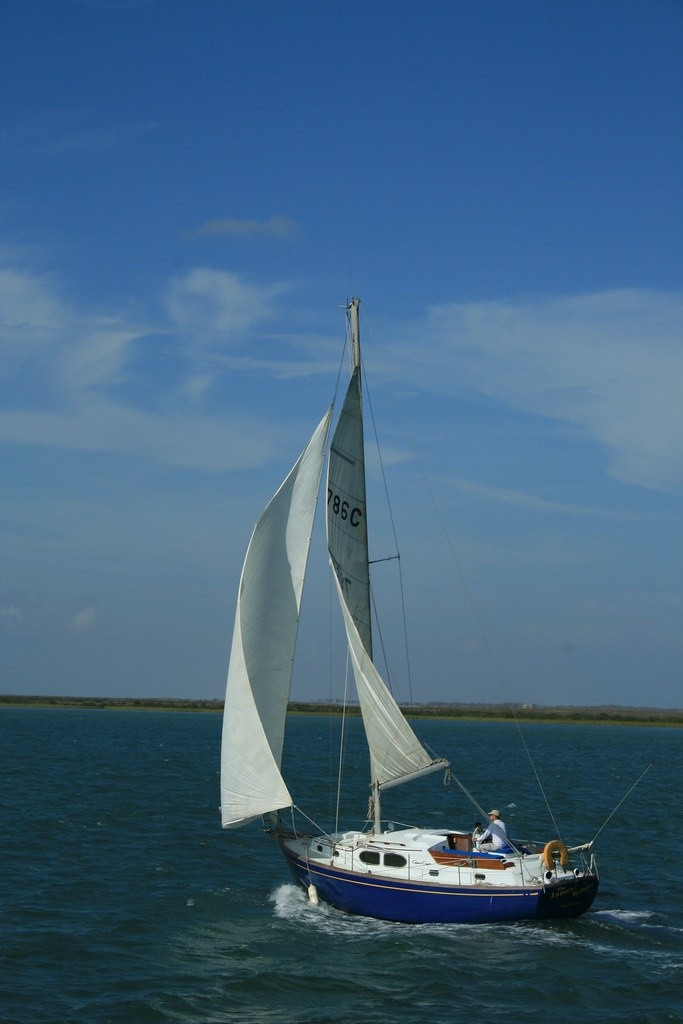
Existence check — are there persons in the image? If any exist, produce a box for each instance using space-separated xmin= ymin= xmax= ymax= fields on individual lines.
xmin=472 ymin=809 xmax=509 ymax=852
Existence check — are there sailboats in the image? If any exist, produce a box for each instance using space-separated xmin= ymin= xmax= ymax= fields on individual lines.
xmin=220 ymin=300 xmax=601 ymax=923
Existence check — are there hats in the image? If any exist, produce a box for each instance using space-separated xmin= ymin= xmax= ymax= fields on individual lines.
xmin=488 ymin=809 xmax=499 ymax=816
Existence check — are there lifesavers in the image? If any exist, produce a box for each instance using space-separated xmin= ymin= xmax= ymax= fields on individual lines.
xmin=543 ymin=840 xmax=567 ymax=869
